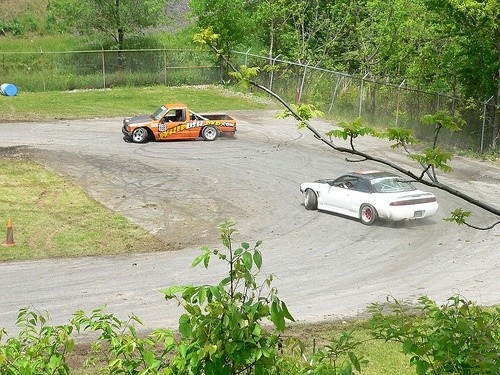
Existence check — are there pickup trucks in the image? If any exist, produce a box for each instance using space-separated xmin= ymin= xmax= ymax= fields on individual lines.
xmin=122 ymin=103 xmax=238 ymax=144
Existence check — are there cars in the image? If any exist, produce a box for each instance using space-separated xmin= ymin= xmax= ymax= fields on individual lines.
xmin=298 ymin=169 xmax=439 ymax=226
